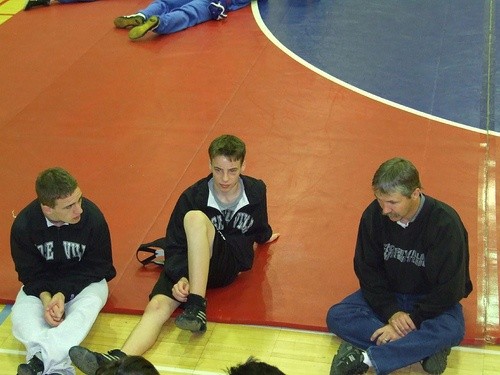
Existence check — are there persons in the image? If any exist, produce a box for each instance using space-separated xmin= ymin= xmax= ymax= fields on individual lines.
xmin=96 ymin=354 xmax=161 ymax=375
xmin=69 ymin=134 xmax=280 ymax=375
xmin=227 ymin=356 xmax=285 ymax=375
xmin=9 ymin=168 xmax=117 ymax=375
xmin=327 ymin=158 xmax=474 ymax=375
xmin=114 ymin=0 xmax=256 ymax=39
xmin=24 ymin=0 xmax=94 ymax=11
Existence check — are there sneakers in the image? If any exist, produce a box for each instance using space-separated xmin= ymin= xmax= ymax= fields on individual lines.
xmin=330 ymin=340 xmax=369 ymax=375
xmin=69 ymin=345 xmax=122 ymax=375
xmin=114 ymin=13 xmax=146 ymax=29
xmin=128 ymin=14 xmax=159 ymax=39
xmin=174 ymin=309 xmax=207 ymax=334
xmin=420 ymin=345 xmax=452 ymax=375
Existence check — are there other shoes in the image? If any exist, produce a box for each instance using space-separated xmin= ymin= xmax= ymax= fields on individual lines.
xmin=18 ymin=357 xmax=44 ymax=375
